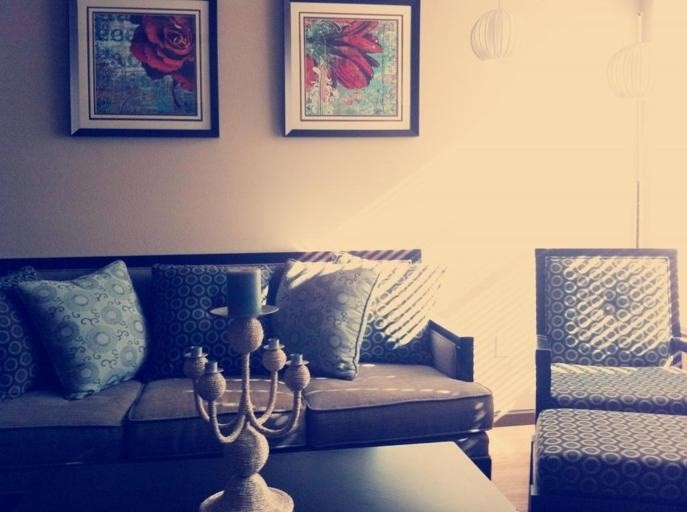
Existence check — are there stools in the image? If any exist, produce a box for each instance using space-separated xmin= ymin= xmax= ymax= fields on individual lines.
xmin=528 ymin=408 xmax=687 ymax=511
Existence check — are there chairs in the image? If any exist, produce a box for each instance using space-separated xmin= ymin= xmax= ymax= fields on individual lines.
xmin=535 ymin=247 xmax=687 ymax=416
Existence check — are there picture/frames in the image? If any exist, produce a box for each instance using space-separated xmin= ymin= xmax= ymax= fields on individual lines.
xmin=282 ymin=0 xmax=420 ymax=136
xmin=69 ymin=0 xmax=220 ymax=138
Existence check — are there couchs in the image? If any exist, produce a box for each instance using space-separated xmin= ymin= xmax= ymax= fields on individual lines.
xmin=2 ymin=249 xmax=495 ymax=481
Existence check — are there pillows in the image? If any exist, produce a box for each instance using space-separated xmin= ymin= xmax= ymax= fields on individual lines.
xmin=3 ymin=265 xmax=55 ymax=400
xmin=271 ymin=257 xmax=383 ymax=381
xmin=16 ymin=259 xmax=150 ymax=400
xmin=325 ymin=250 xmax=446 ymax=367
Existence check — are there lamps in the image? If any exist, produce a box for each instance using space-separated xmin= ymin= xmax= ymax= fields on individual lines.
xmin=183 ymin=264 xmax=312 ymax=512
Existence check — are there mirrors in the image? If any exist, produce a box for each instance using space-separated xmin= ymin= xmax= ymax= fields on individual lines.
xmin=146 ymin=264 xmax=277 ymax=381
